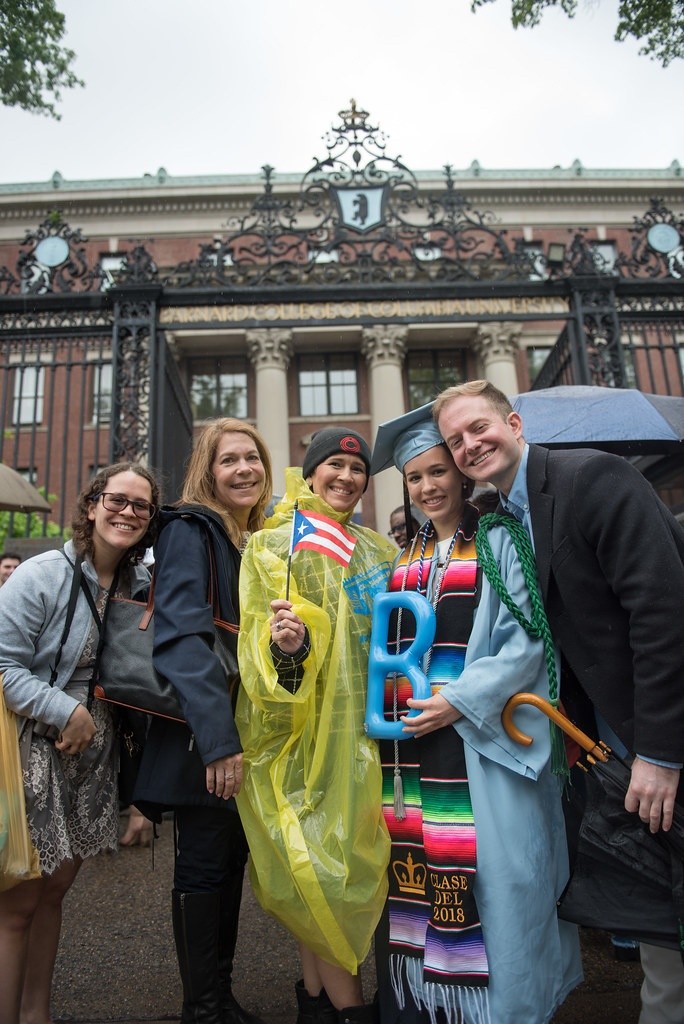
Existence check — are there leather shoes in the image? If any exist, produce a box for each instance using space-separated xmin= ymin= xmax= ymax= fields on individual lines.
xmin=600 ymin=931 xmax=641 ymax=957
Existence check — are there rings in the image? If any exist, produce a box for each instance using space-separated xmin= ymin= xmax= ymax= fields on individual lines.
xmin=276 ymin=622 xmax=283 ymax=630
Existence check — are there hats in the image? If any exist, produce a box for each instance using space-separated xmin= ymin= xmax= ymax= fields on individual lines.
xmin=371 ymin=400 xmax=445 ymax=476
xmin=303 ymin=428 xmax=370 ymax=479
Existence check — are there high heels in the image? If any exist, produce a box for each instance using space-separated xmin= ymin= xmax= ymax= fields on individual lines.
xmin=118 ymin=816 xmax=152 ymax=846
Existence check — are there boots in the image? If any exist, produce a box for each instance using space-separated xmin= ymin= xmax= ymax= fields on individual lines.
xmin=171 ymin=891 xmax=264 ymax=1024
xmin=295 ymin=980 xmax=341 ymax=1024
xmin=338 ymin=1005 xmax=381 ymax=1024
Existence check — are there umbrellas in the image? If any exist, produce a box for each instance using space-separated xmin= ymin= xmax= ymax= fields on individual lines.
xmin=501 ymin=692 xmax=683 ymax=951
xmin=510 ymin=385 xmax=684 ymax=455
xmin=0 ymin=463 xmax=51 ymax=514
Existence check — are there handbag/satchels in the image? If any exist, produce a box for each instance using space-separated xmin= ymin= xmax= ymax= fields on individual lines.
xmin=93 ymin=598 xmax=239 ymax=723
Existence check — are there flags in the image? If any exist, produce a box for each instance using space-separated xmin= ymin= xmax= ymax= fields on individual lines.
xmin=293 ymin=509 xmax=358 ymax=568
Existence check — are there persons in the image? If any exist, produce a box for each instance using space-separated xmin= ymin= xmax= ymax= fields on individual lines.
xmin=118 ymin=416 xmax=273 ymax=1024
xmin=233 ymin=425 xmax=402 ymax=1024
xmin=429 ymin=380 xmax=684 ymax=1024
xmin=472 ymin=489 xmax=500 ymax=515
xmin=0 ymin=552 xmax=21 ymax=585
xmin=368 ymin=399 xmax=585 ymax=1024
xmin=0 ymin=461 xmax=166 ymax=1024
xmin=388 ymin=504 xmax=420 ymax=550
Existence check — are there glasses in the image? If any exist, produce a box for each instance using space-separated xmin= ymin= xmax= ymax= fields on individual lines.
xmin=102 ymin=493 xmax=156 ymax=520
xmin=388 ymin=524 xmax=406 ymax=538
